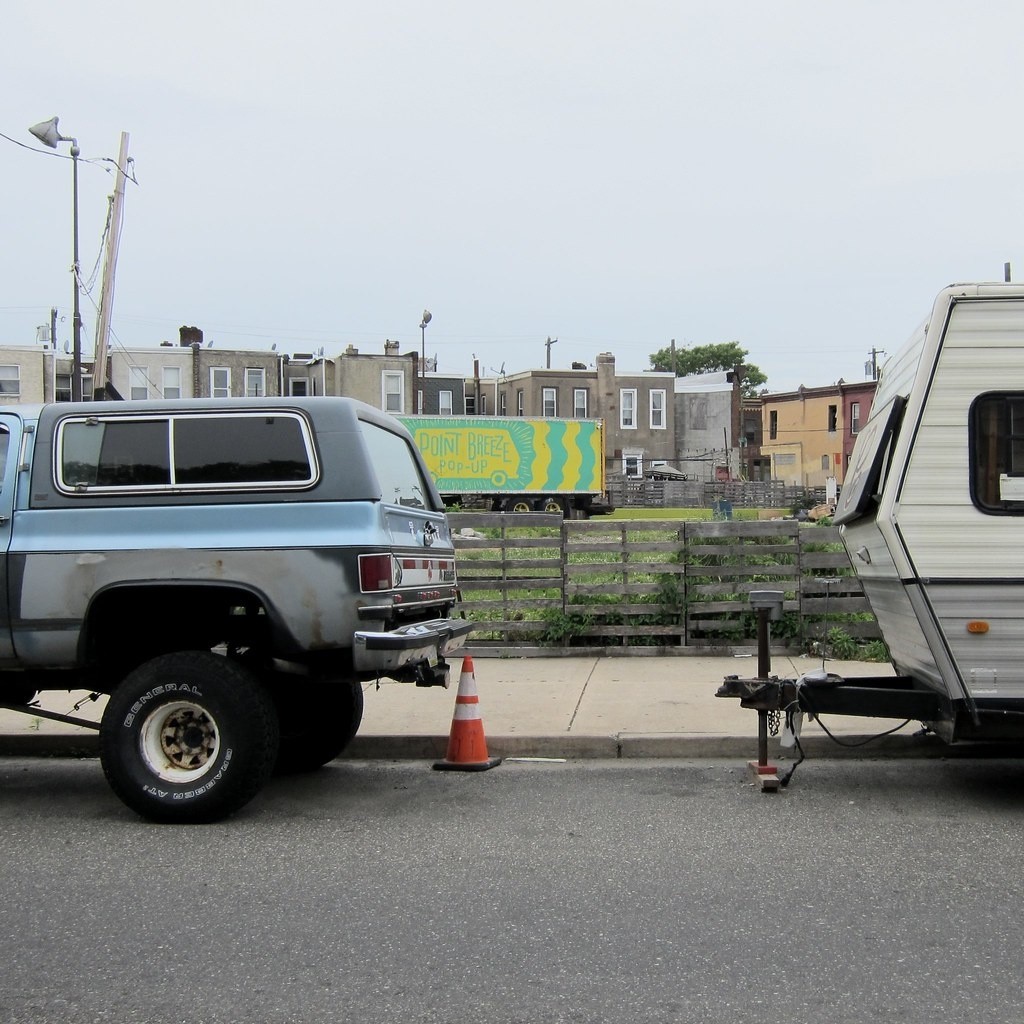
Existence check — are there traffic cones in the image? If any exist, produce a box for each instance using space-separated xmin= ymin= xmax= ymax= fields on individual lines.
xmin=428 ymin=656 xmax=503 ymax=771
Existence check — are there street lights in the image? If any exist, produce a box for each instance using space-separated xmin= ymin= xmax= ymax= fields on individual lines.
xmin=28 ymin=115 xmax=82 ymax=403
xmin=419 ymin=311 xmax=432 ymax=415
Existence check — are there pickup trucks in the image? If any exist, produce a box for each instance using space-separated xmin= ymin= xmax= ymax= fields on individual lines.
xmin=1 ymin=395 xmax=476 ymax=826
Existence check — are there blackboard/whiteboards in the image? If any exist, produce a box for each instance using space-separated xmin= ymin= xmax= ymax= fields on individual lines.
xmin=825 ymin=478 xmax=838 ymax=506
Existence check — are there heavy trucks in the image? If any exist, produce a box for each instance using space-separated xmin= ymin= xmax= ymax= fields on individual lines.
xmin=388 ymin=412 xmax=615 ymax=519
